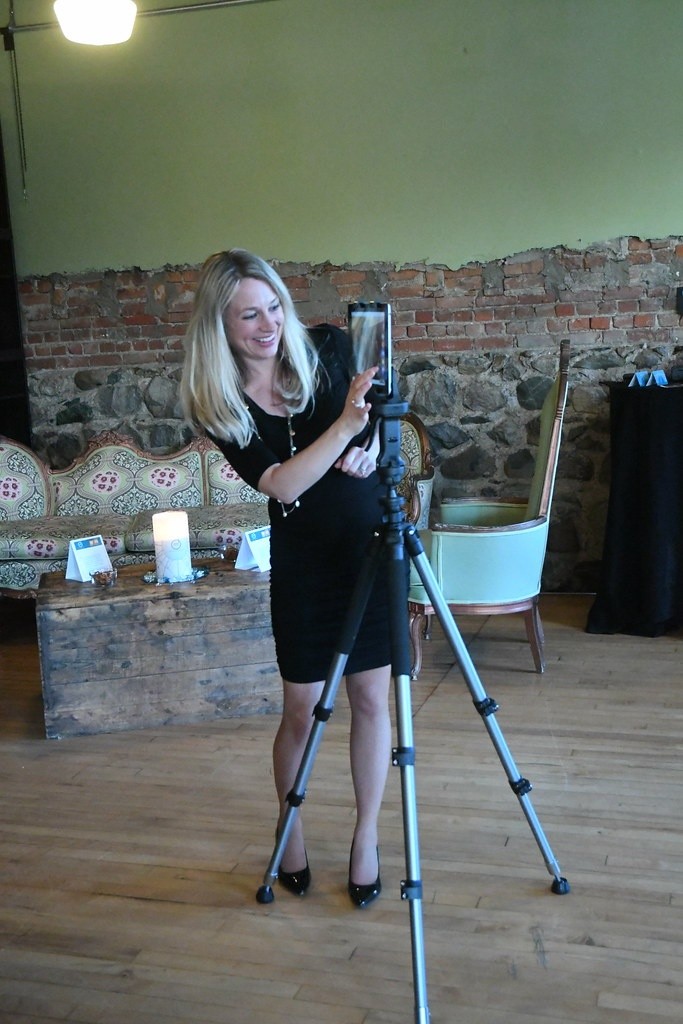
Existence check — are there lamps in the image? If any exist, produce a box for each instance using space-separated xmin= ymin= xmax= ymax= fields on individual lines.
xmin=143 ymin=510 xmax=210 ymax=587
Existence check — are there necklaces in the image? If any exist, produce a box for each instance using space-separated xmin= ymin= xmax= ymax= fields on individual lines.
xmin=244 ymin=401 xmax=301 ymax=518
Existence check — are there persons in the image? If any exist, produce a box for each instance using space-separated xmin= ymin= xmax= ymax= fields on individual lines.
xmin=177 ymin=245 xmax=395 ymax=909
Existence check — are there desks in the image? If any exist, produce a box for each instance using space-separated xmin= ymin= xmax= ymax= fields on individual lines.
xmin=36 ymin=558 xmax=284 ymax=739
xmin=585 ymin=382 xmax=683 ymax=637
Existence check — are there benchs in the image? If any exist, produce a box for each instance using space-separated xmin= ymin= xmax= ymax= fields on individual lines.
xmin=0 ymin=411 xmax=434 ymax=599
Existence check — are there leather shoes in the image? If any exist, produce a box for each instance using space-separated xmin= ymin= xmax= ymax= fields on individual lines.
xmin=348 ymin=826 xmax=381 ymax=906
xmin=275 ymin=828 xmax=312 ymax=895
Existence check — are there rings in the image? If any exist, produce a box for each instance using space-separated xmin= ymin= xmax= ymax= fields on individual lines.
xmin=350 ymin=399 xmax=365 ymax=408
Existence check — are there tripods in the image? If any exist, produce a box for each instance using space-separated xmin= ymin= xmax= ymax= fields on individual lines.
xmin=255 ymin=367 xmax=571 ymax=1024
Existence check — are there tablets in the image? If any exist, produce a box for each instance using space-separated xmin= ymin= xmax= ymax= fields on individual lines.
xmin=348 ymin=300 xmax=391 ymax=394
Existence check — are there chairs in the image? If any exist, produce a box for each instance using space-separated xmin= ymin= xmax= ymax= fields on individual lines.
xmin=409 ymin=340 xmax=572 ymax=682
